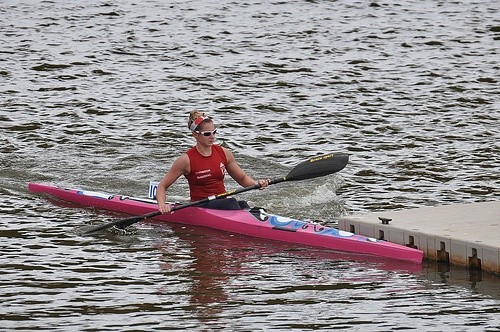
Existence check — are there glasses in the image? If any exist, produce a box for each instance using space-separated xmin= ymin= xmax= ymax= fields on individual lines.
xmin=195 ymin=127 xmax=217 ymax=137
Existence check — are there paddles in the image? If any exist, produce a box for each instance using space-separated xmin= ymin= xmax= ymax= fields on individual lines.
xmin=75 ymin=150 xmax=351 ymax=237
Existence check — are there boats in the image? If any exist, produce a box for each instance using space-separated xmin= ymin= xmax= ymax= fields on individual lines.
xmin=28 ymin=182 xmax=423 ymax=264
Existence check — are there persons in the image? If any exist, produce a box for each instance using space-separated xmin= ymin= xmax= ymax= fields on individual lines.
xmin=156 ymin=111 xmax=268 ymax=215
